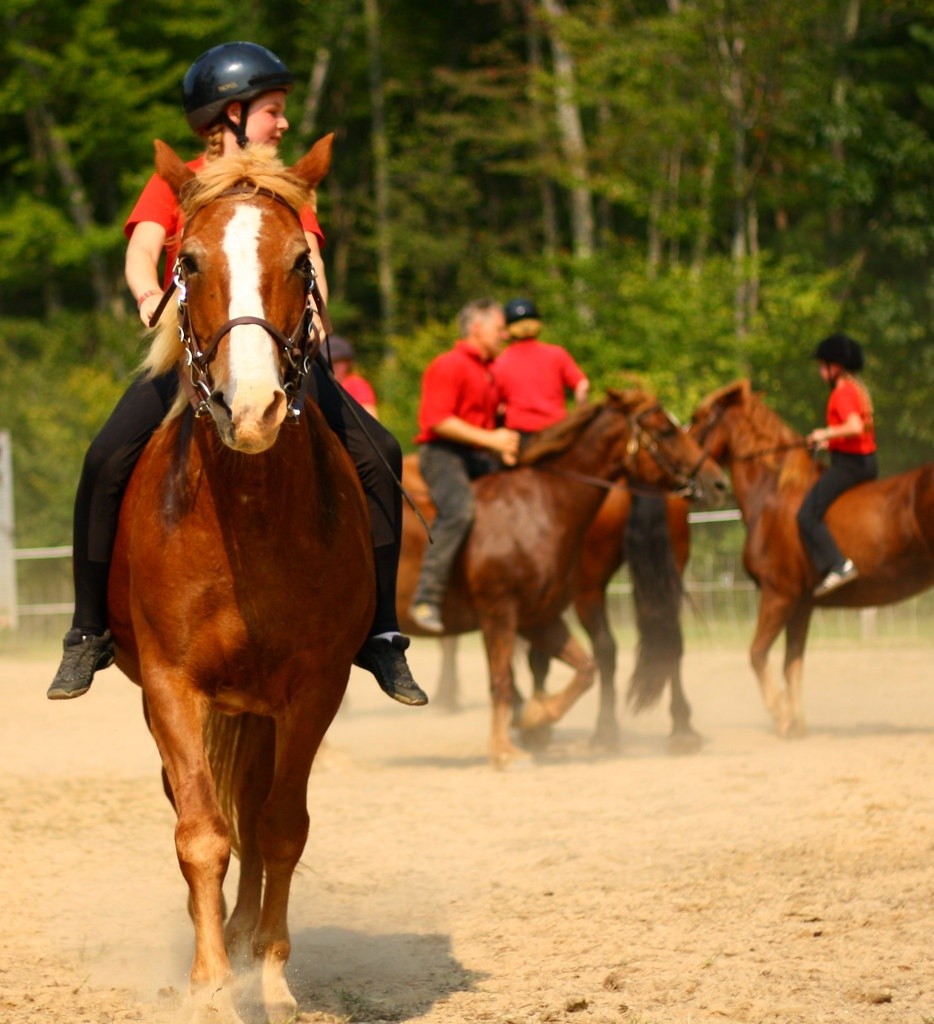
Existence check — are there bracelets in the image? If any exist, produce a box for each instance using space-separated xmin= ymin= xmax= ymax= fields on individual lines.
xmin=137 ymin=290 xmax=164 ymax=310
xmin=826 ymin=428 xmax=830 ymax=438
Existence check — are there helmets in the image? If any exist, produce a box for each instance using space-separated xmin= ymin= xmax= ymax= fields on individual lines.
xmin=808 ymin=335 xmax=863 ymax=370
xmin=506 ymin=299 xmax=541 ymax=325
xmin=182 ymin=41 xmax=294 ymax=135
xmin=320 ymin=335 xmax=354 ymax=363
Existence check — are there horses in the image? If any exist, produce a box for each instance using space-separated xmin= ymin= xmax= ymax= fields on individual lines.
xmin=395 ymin=378 xmax=934 ymax=772
xmin=108 ymin=132 xmax=378 ymax=1024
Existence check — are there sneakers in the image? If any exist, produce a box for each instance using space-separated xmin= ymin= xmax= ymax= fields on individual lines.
xmin=47 ymin=628 xmax=115 ymax=699
xmin=353 ymin=635 xmax=428 ymax=706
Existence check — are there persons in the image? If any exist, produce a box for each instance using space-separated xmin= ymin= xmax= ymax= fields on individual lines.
xmin=321 ymin=299 xmax=589 ymax=633
xmin=46 ymin=42 xmax=430 ymax=707
xmin=796 ymin=332 xmax=879 ymax=597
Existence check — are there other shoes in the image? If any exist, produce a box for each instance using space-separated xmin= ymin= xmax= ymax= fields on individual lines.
xmin=812 ymin=559 xmax=858 ymax=597
xmin=407 ymin=602 xmax=445 ymax=633
